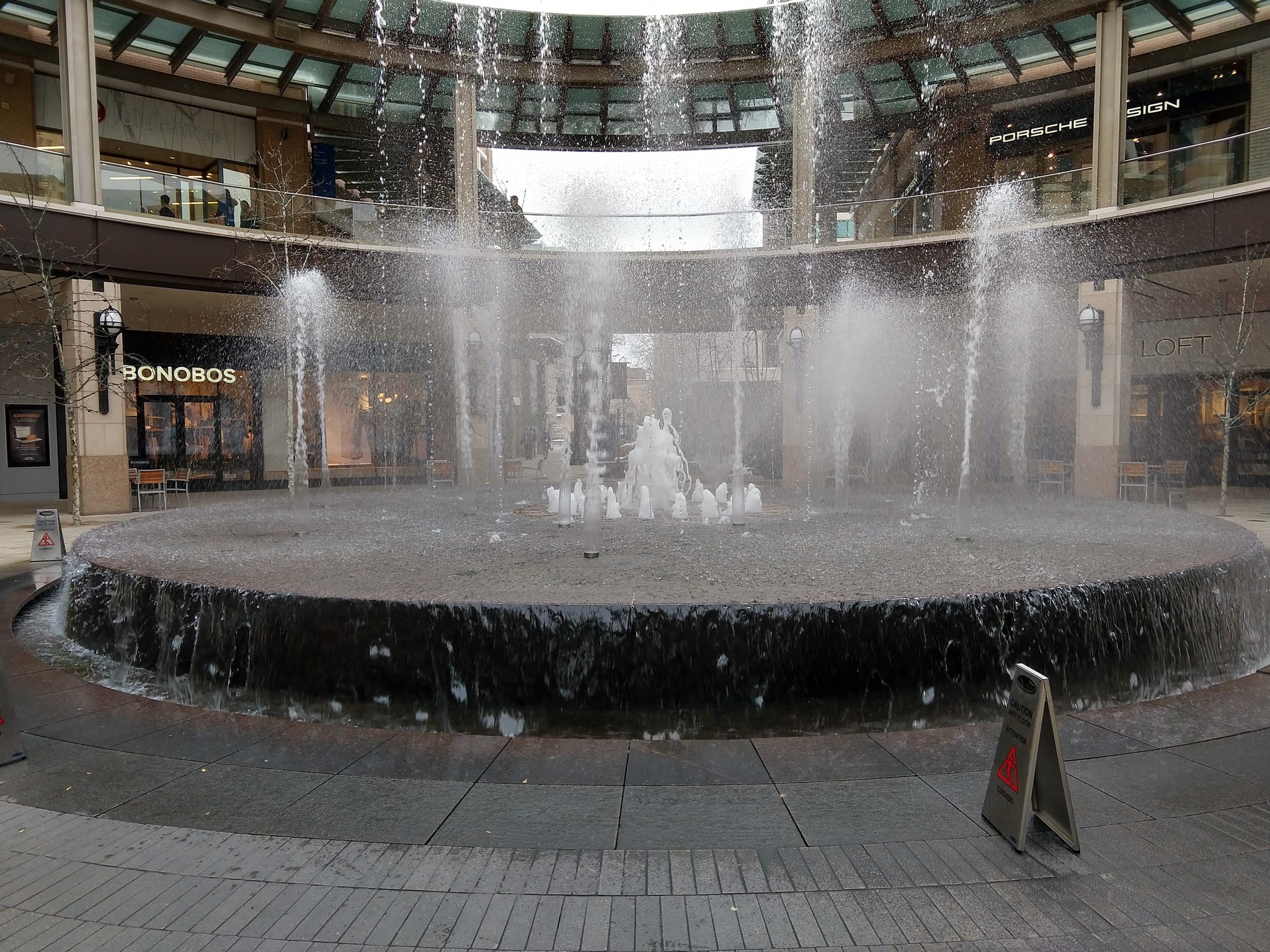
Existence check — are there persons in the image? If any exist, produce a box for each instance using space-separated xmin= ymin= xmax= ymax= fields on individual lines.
xmin=214 ymin=189 xmax=239 ymax=227
xmin=239 ymin=200 xmax=261 ymax=229
xmin=159 ymin=195 xmax=176 ymax=218
xmin=520 ymin=426 xmax=554 ymax=461
xmin=503 ymin=196 xmax=525 ymax=252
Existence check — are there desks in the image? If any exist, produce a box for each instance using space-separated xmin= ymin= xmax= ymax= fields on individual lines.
xmin=1149 ymin=466 xmax=1162 ymax=503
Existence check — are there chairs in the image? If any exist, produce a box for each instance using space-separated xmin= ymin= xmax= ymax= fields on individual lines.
xmin=503 ymin=459 xmax=524 ymax=486
xmin=427 ymin=460 xmax=454 ymax=488
xmin=128 ymin=468 xmax=140 ymax=509
xmin=1029 ymin=459 xmax=1074 ymax=495
xmin=1118 ymin=460 xmax=1188 ymax=504
xmin=137 ymin=469 xmax=166 ymax=513
xmin=165 ymin=468 xmax=191 ymax=508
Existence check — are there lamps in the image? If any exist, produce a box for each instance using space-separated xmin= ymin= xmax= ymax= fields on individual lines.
xmin=786 ymin=325 xmax=808 ymax=414
xmin=1076 ymin=304 xmax=1104 ymax=407
xmin=273 ymin=20 xmax=300 ymax=42
xmin=93 ymin=305 xmax=130 ymax=415
xmin=465 ymin=328 xmax=483 ymax=414
